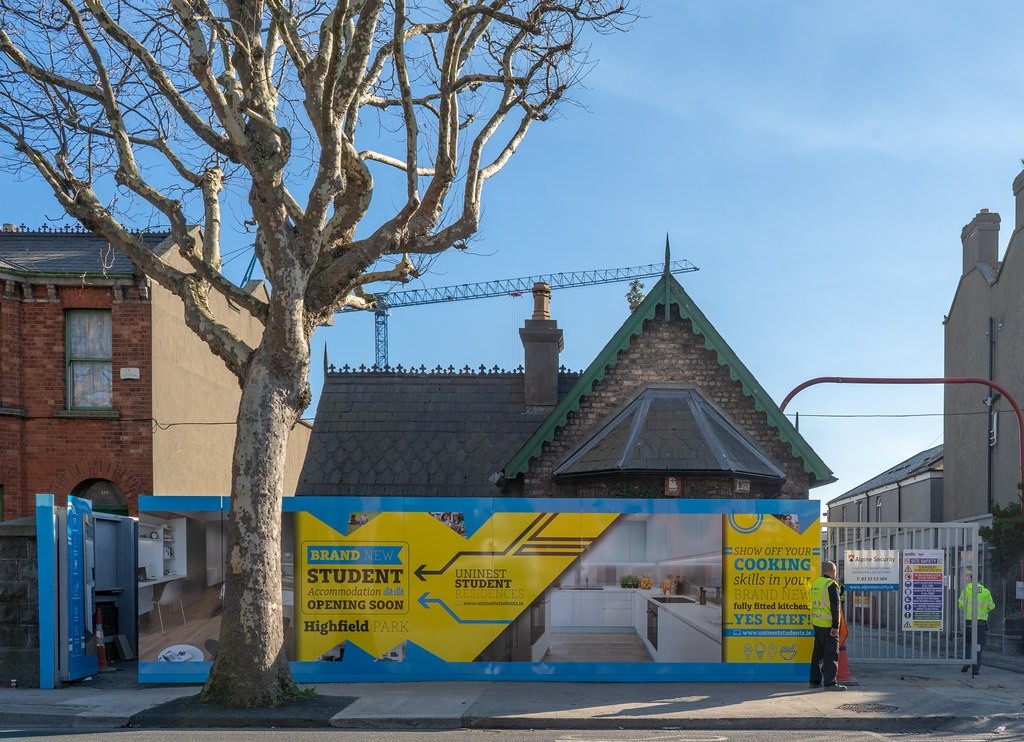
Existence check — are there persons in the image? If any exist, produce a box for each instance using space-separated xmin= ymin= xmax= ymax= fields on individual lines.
xmin=808 ymin=558 xmax=849 ymax=692
xmin=837 ymin=578 xmax=846 ymax=618
xmin=957 ymin=572 xmax=996 ymax=677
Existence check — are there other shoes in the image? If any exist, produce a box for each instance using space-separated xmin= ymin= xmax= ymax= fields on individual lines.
xmin=972 ymin=664 xmax=978 ymax=675
xmin=808 ymin=683 xmax=824 ymax=688
xmin=961 ymin=665 xmax=970 ymax=673
xmin=823 ymin=683 xmax=848 ymax=692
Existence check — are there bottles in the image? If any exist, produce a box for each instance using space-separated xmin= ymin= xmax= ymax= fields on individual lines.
xmin=664 ymin=575 xmax=707 ymax=606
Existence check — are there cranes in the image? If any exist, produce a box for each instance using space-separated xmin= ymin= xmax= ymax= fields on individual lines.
xmin=331 ymin=257 xmax=700 ymax=371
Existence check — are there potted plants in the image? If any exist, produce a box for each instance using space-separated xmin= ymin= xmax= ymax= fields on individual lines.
xmin=632 ymin=576 xmax=640 ymax=588
xmin=619 ymin=575 xmax=627 ymax=588
xmin=626 ymin=575 xmax=633 ymax=588
